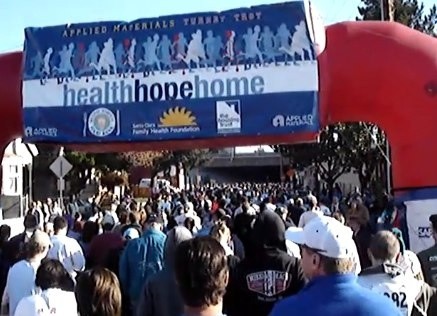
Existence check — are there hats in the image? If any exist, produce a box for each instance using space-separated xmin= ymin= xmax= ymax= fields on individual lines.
xmin=23 ymin=215 xmax=37 ymax=228
xmin=252 ymin=210 xmax=285 ymax=249
xmin=145 ymin=213 xmax=163 ymax=224
xmin=284 ymin=216 xmax=354 ymax=258
xmin=121 ymin=228 xmax=139 ymax=242
xmin=100 ymin=215 xmax=114 ymax=227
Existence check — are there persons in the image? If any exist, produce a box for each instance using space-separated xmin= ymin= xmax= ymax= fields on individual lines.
xmin=1 ymin=173 xmax=436 ymax=315
